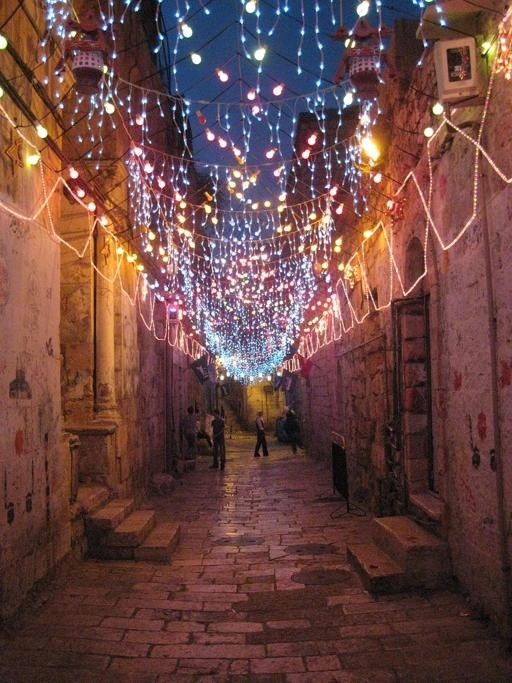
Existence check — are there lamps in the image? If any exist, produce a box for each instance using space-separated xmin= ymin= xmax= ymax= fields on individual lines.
xmin=62 ymin=8 xmax=110 ymax=96
xmin=347 ymin=15 xmax=383 ymax=93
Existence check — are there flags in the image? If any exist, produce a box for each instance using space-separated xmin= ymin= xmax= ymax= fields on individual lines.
xmin=273 ymin=375 xmax=282 ymax=391
xmin=207 ymin=363 xmax=218 ymax=384
xmin=299 ymin=354 xmax=313 ymax=379
xmin=190 ymin=355 xmax=208 ymax=383
xmin=281 ymin=369 xmax=297 ymax=393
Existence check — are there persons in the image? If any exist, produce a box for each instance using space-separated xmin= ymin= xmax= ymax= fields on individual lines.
xmin=183 ymin=404 xmax=202 ymax=456
xmin=253 ymin=410 xmax=268 ymax=456
xmin=209 ymin=408 xmax=226 ymax=471
xmin=281 ymin=405 xmax=299 ymax=454
